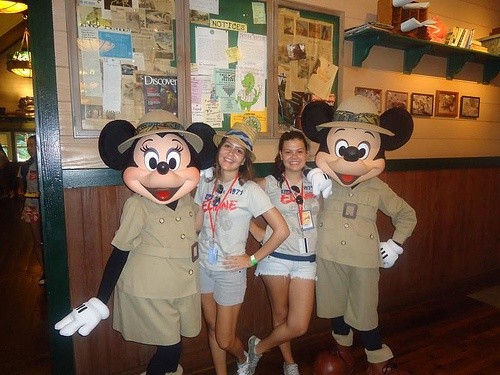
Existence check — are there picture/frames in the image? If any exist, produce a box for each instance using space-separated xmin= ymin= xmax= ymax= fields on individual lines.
xmin=410 ymin=92 xmax=434 ymax=116
xmin=435 ymin=90 xmax=459 ymax=117
xmin=460 ymin=95 xmax=481 ymax=118
xmin=385 ymin=90 xmax=408 ymax=112
xmin=355 ymin=86 xmax=383 ymax=113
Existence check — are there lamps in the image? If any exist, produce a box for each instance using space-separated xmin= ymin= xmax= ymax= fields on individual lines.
xmin=0 ymin=0 xmax=33 ymax=80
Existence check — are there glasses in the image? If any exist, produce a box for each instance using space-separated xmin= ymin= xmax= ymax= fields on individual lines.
xmin=291 ymin=185 xmax=304 ymax=205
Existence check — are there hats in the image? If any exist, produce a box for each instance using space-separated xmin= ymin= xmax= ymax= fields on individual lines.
xmin=214 ymin=122 xmax=256 ymax=163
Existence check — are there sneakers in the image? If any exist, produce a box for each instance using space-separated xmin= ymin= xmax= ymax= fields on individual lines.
xmin=246 ymin=336 xmax=261 ymax=375
xmin=238 ymin=351 xmax=249 ymax=375
xmin=283 ymin=361 xmax=298 ymax=375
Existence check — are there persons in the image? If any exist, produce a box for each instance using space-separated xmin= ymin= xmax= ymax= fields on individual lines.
xmin=248 ymin=131 xmax=318 ymax=375
xmin=17 ymin=136 xmax=48 ymax=286
xmin=193 ymin=122 xmax=290 ymax=375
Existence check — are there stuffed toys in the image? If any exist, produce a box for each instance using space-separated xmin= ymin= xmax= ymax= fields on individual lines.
xmin=301 ymin=101 xmax=418 ymax=375
xmin=54 ymin=109 xmax=217 ymax=375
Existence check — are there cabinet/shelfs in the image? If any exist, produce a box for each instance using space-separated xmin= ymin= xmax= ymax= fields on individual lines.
xmin=376 ymin=0 xmax=428 ymax=39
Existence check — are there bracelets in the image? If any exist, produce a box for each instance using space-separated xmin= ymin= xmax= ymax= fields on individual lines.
xmin=250 ymin=255 xmax=257 ymax=265
